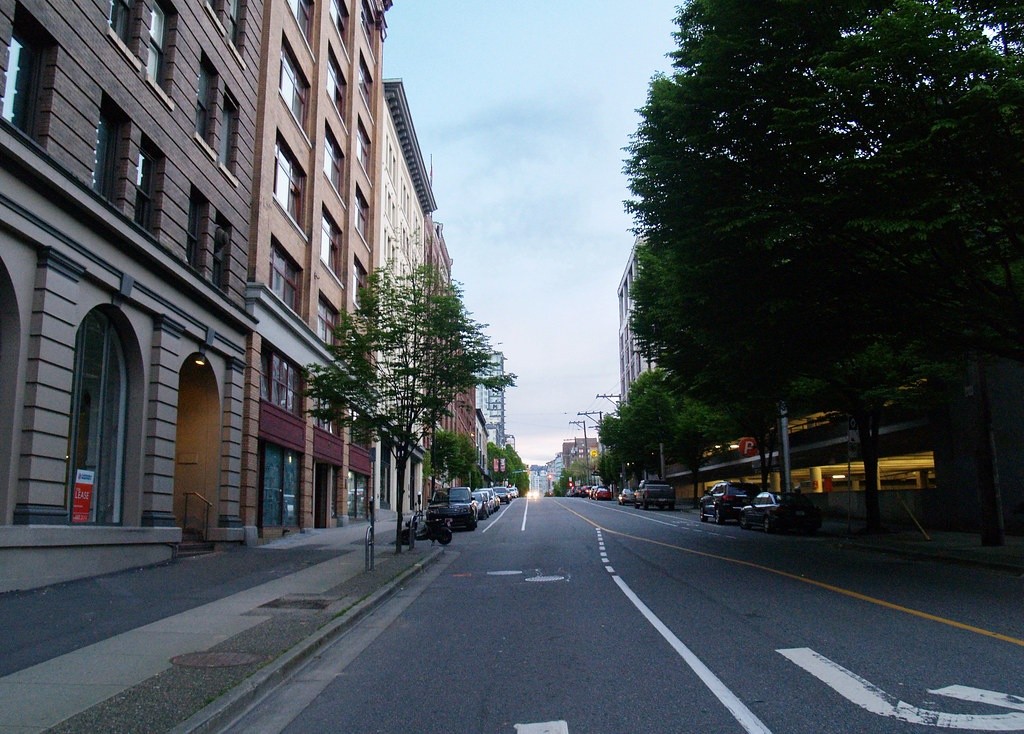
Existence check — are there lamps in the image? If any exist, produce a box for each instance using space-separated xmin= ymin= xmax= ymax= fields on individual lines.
xmin=195 ymin=354 xmax=205 ymax=365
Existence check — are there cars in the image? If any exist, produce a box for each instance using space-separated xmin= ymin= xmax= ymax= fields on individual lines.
xmin=619 ymin=489 xmax=636 ymax=506
xmin=566 ymin=485 xmax=612 ymax=500
xmin=738 ymin=491 xmax=822 ymax=536
xmin=471 ymin=487 xmax=520 ymax=520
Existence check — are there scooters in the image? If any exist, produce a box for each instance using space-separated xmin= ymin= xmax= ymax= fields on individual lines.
xmin=401 ymin=509 xmax=453 ymax=545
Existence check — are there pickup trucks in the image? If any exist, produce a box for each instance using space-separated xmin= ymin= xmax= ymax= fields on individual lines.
xmin=634 ymin=479 xmax=675 ymax=511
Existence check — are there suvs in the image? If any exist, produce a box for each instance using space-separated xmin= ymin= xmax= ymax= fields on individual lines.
xmin=425 ymin=486 xmax=477 ymax=530
xmin=700 ymin=481 xmax=762 ymax=524
